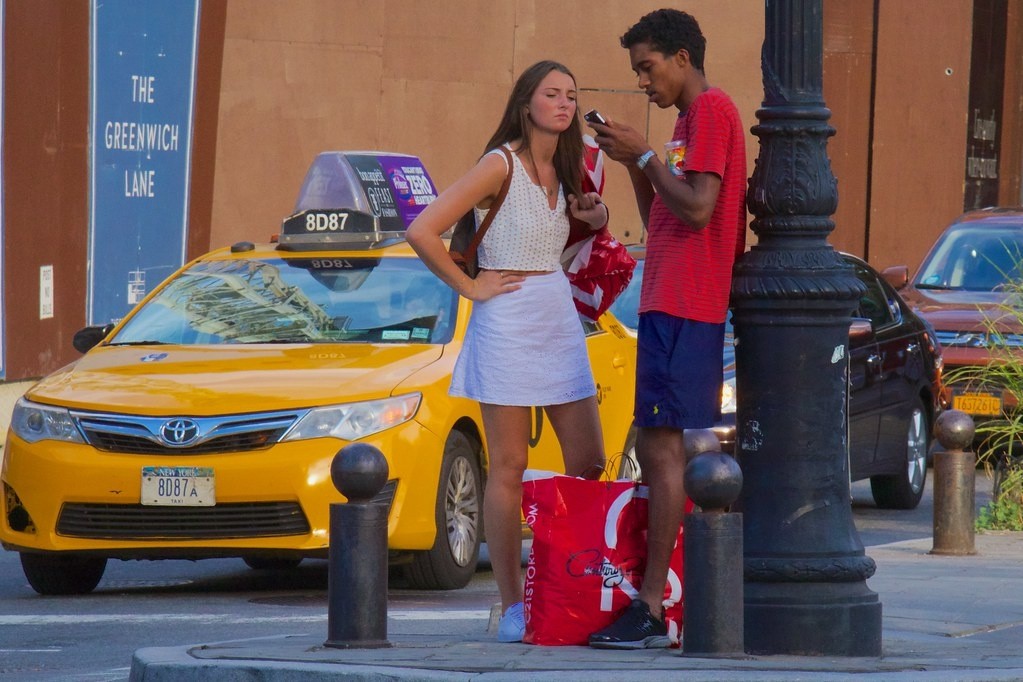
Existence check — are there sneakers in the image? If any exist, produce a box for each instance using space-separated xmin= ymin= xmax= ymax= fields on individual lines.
xmin=496 ymin=602 xmax=525 ymax=642
xmin=588 ymin=599 xmax=670 ymax=650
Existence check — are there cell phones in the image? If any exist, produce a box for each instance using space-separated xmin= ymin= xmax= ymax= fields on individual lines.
xmin=584 ymin=109 xmax=610 ymax=138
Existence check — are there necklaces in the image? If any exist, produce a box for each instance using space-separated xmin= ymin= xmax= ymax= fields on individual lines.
xmin=538 ymin=161 xmax=556 ymax=196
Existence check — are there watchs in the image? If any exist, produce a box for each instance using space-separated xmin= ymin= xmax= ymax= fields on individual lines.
xmin=636 ymin=151 xmax=657 ymax=169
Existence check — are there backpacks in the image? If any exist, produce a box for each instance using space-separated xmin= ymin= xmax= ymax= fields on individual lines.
xmin=445 ymin=145 xmax=512 ymax=278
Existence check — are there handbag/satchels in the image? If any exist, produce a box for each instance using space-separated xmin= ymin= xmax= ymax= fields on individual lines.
xmin=559 ymin=134 xmax=636 ymax=319
xmin=521 ymin=452 xmax=695 ymax=648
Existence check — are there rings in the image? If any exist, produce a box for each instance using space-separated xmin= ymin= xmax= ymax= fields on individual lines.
xmin=498 ymin=272 xmax=504 ymax=278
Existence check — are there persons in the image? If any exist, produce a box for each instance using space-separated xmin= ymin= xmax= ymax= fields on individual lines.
xmin=588 ymin=5 xmax=747 ymax=650
xmin=406 ymin=59 xmax=608 ymax=642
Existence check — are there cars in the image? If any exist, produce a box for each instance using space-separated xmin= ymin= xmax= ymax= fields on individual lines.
xmin=607 ymin=242 xmax=938 ymax=510
xmin=882 ymin=207 xmax=1023 ymax=459
xmin=0 ymin=152 xmax=638 ymax=596
